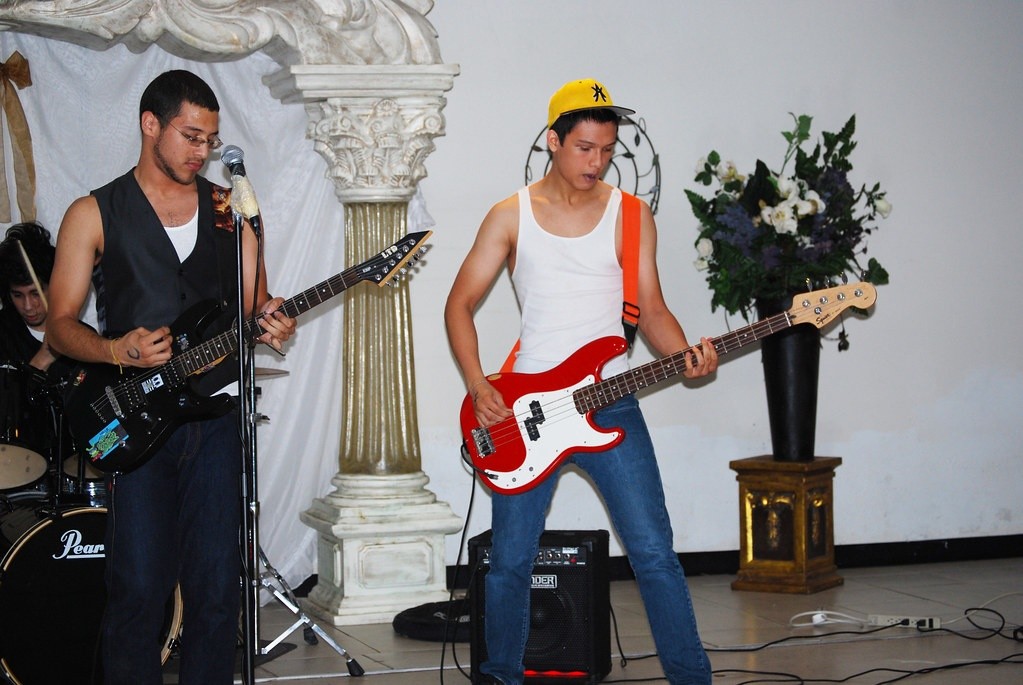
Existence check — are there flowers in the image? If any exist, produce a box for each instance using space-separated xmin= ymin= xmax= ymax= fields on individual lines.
xmin=684 ymin=111 xmax=893 ymax=352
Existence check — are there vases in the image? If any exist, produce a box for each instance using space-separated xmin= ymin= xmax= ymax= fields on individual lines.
xmin=753 ymin=284 xmax=820 ymax=462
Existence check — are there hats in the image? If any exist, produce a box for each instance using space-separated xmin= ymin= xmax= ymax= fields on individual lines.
xmin=547 ymin=78 xmax=636 ymax=129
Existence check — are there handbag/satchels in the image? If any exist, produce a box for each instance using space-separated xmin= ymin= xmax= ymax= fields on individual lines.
xmin=393 ymin=600 xmax=472 ymax=643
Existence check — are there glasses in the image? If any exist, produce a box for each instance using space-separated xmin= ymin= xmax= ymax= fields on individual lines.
xmin=151 ymin=112 xmax=223 ymax=149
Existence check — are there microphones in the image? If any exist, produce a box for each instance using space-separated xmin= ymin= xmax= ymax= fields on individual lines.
xmin=221 ymin=145 xmax=263 ymax=236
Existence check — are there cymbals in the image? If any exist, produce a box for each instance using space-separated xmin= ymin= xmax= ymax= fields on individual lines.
xmin=251 ymin=368 xmax=289 ymax=381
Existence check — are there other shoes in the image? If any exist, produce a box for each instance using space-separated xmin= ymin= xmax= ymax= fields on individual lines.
xmin=479 ymin=673 xmax=505 ymax=685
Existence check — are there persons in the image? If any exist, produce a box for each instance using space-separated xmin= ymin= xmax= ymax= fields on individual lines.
xmin=444 ymin=79 xmax=719 ymax=685
xmin=0 ymin=69 xmax=298 ymax=685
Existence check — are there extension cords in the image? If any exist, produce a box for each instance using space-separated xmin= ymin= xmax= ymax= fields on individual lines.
xmin=868 ymin=614 xmax=940 ymax=628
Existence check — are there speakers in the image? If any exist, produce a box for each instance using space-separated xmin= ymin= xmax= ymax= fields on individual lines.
xmin=467 ymin=528 xmax=611 ymax=685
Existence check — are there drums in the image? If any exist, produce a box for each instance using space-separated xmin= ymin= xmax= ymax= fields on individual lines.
xmin=0 ymin=358 xmax=59 ymax=492
xmin=46 ymin=365 xmax=108 ymax=481
xmin=0 ymin=491 xmax=183 ymax=685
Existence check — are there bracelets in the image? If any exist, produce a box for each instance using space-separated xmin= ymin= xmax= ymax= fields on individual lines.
xmin=110 ymin=338 xmax=123 ymax=375
xmin=469 ymin=380 xmax=487 ymax=390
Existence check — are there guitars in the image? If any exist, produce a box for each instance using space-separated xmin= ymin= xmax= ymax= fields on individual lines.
xmin=62 ymin=227 xmax=433 ymax=479
xmin=460 ymin=269 xmax=878 ymax=494
